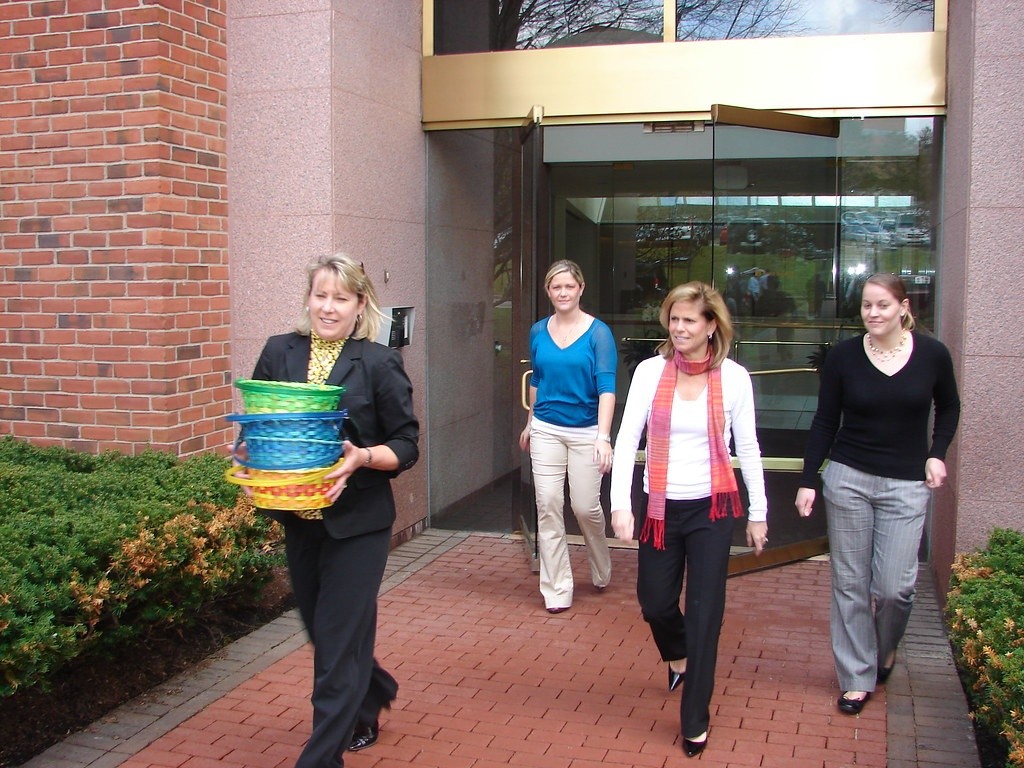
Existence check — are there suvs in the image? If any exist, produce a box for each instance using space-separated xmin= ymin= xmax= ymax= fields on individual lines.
xmin=727 ymin=217 xmax=769 ymax=253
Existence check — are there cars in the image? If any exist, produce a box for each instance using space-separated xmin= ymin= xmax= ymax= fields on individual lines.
xmin=881 ymin=210 xmax=900 ymax=218
xmin=720 ymin=224 xmax=727 ymax=245
xmin=841 ymin=221 xmax=895 ymax=246
xmin=672 ymin=216 xmax=711 ymax=245
xmin=879 ymin=218 xmax=895 ymax=246
xmin=841 ymin=210 xmax=882 ymax=231
xmin=895 ymin=212 xmax=931 ymax=246
xmin=707 ymin=216 xmax=742 ymax=235
xmin=635 ymin=218 xmax=667 ymax=245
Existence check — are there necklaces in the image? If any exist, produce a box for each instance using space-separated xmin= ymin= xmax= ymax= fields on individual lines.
xmin=867 ymin=328 xmax=907 ymax=362
xmin=561 ymin=331 xmax=571 ymax=341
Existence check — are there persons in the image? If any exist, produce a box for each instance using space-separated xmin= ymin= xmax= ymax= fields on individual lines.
xmin=812 ymin=272 xmax=825 ymax=318
xmin=520 ymin=259 xmax=618 ymax=614
xmin=794 ymin=274 xmax=961 ymax=714
xmin=610 ymin=280 xmax=769 ymax=756
xmin=723 ymin=268 xmax=779 ymax=313
xmin=226 ymin=252 xmax=419 ymax=768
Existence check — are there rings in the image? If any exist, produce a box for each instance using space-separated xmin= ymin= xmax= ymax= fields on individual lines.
xmin=762 ymin=538 xmax=768 ymax=542
xmin=941 ymin=483 xmax=943 ymax=486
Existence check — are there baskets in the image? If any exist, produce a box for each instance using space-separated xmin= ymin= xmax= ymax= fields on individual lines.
xmin=225 ymin=377 xmax=352 ymax=510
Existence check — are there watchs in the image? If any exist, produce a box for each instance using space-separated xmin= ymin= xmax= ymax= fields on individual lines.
xmin=364 ymin=448 xmax=372 ymax=467
xmin=596 ymin=434 xmax=611 ymax=442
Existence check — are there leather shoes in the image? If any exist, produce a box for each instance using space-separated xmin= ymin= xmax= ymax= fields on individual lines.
xmin=838 ymin=690 xmax=871 ymax=714
xmin=349 ymin=683 xmax=398 ymax=750
xmin=682 ymin=736 xmax=709 ymax=756
xmin=593 ymin=583 xmax=606 ymax=591
xmin=668 ymin=660 xmax=685 ymax=691
xmin=876 ymin=646 xmax=896 ymax=682
xmin=548 ymin=607 xmax=567 ymax=614
